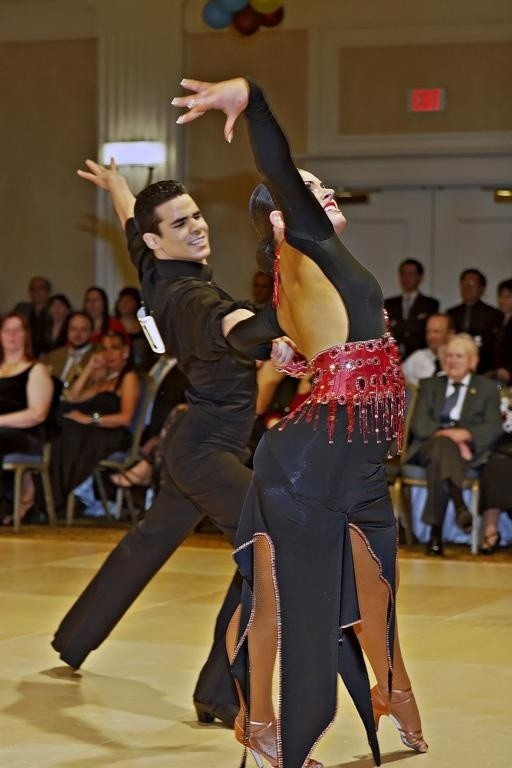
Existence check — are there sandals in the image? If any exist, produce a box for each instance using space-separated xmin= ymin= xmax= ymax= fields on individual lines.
xmin=109 ymin=470 xmax=150 ymax=489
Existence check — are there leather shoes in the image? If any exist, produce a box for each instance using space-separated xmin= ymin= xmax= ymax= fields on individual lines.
xmin=457 ymin=508 xmax=471 ymax=533
xmin=426 ymin=540 xmax=443 ymax=556
xmin=193 ymin=698 xmax=238 ymax=729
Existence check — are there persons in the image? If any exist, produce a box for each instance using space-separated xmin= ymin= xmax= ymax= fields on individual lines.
xmin=49 ymin=154 xmax=284 ymax=732
xmin=1 ymin=258 xmax=512 ymax=563
xmin=168 ymin=73 xmax=429 ymax=768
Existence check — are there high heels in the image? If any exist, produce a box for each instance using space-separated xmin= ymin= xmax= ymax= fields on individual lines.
xmin=234 ymin=705 xmax=323 ymax=767
xmin=371 ymin=684 xmax=428 ymax=752
xmin=479 ymin=531 xmax=501 ymax=553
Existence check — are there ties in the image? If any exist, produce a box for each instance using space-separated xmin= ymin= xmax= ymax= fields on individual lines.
xmin=403 ymin=297 xmax=409 ymax=319
xmin=66 ymin=353 xmax=78 ymax=382
xmin=441 ymin=381 xmax=463 ymax=427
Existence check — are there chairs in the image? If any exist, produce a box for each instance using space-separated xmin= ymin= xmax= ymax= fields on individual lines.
xmin=65 ymin=373 xmax=157 ymax=535
xmin=387 ymin=379 xmax=414 ymax=552
xmin=397 ymin=461 xmax=488 ymax=556
xmin=2 ymin=391 xmax=69 ymax=533
